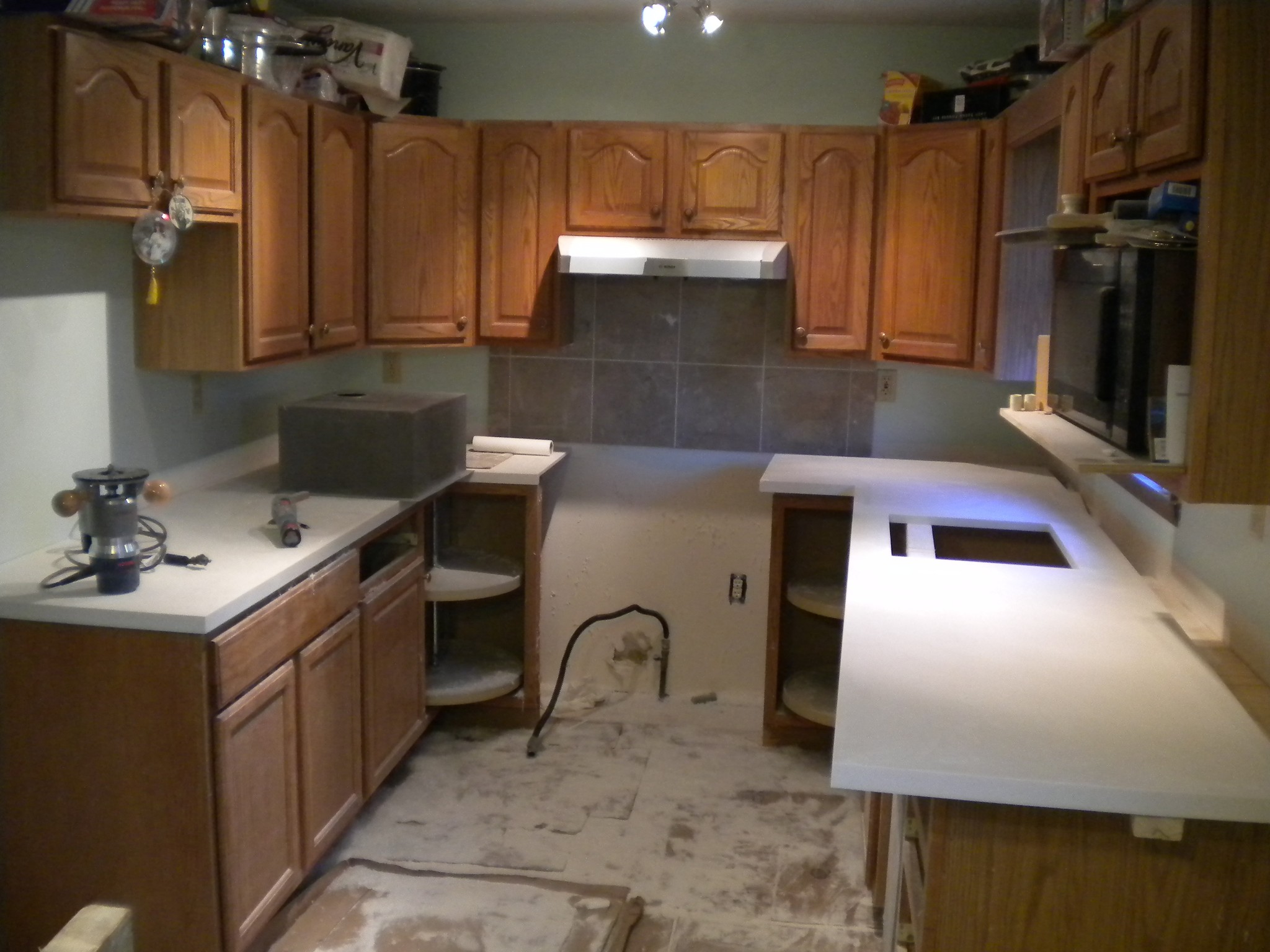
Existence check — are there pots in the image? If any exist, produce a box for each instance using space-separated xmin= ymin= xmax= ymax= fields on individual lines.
xmin=400 ymin=61 xmax=446 ymax=117
xmin=57 ymin=0 xmax=329 ymax=91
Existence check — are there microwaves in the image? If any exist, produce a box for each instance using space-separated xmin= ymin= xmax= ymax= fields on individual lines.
xmin=1047 ymin=244 xmax=1195 ymax=454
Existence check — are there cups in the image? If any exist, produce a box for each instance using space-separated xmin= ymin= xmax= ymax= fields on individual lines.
xmin=1024 ymin=394 xmax=1036 ymax=411
xmin=1010 ymin=393 xmax=1022 ymax=411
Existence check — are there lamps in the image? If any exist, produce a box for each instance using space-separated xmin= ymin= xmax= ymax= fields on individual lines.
xmin=641 ymin=0 xmax=724 ymax=34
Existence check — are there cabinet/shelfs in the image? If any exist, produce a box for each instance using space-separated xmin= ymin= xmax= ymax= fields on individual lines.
xmin=0 ymin=485 xmax=542 ymax=952
xmin=785 ymin=124 xmax=884 ymax=352
xmin=871 ymin=117 xmax=1005 ymax=369
xmin=136 ymin=85 xmax=367 ymax=370
xmin=1 ymin=13 xmax=241 ymax=223
xmin=762 ymin=490 xmax=1270 ymax=952
xmin=368 ymin=115 xmax=475 ymax=349
xmin=474 ymin=120 xmax=567 ymax=346
xmin=564 ymin=120 xmax=786 ymax=237
xmin=1059 ymin=0 xmax=1269 ymax=502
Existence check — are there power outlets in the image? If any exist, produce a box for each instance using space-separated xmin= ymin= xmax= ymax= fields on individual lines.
xmin=732 ymin=577 xmax=744 ymax=599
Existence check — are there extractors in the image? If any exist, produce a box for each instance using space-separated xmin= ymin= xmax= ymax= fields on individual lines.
xmin=557 ymin=236 xmax=789 ymax=281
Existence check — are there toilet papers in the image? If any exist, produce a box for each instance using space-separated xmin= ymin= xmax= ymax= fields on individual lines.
xmin=471 ymin=435 xmax=554 ymax=456
